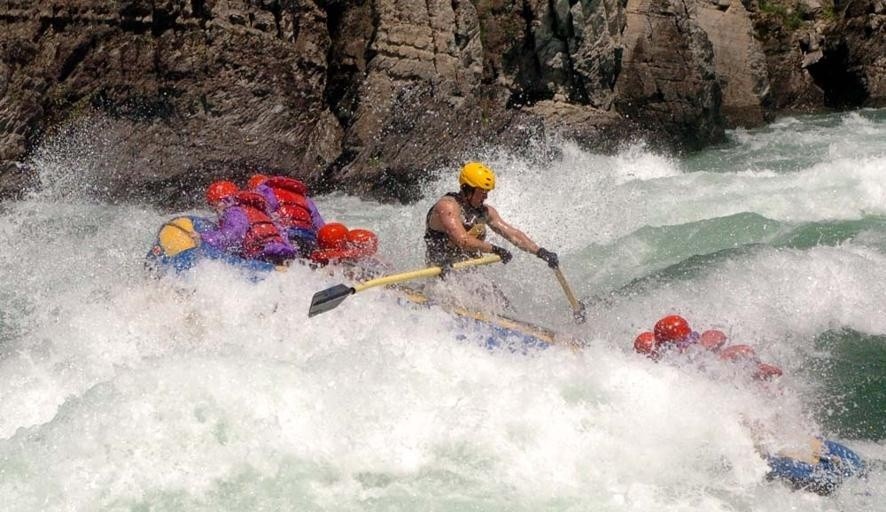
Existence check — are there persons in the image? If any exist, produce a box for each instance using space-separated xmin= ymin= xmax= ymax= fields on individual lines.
xmin=633 ymin=320 xmax=783 ymax=396
xmin=246 ymin=174 xmax=325 ymax=258
xmin=350 ymin=230 xmax=383 ymax=276
xmin=313 ymin=223 xmax=361 ymax=277
xmin=423 ymin=162 xmax=559 ymax=321
xmin=190 ymin=179 xmax=301 ymax=270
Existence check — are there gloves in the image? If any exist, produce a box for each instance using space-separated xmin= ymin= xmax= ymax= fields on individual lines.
xmin=536 ymin=248 xmax=559 ymax=268
xmin=490 ymin=243 xmax=512 ymax=265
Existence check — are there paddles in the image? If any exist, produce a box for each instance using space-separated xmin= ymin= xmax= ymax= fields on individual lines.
xmin=308 ymin=253 xmax=501 ymax=317
xmin=554 ymin=267 xmax=586 ymax=324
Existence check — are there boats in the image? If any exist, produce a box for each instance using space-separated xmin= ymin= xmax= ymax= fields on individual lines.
xmin=142 ymin=215 xmax=865 ymax=494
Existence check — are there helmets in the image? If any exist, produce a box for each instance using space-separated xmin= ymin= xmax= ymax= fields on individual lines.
xmin=207 ymin=181 xmax=239 ymax=203
xmin=634 ymin=333 xmax=660 ymax=358
xmin=459 ymin=163 xmax=495 ymax=190
xmin=248 ymin=176 xmax=266 ymax=191
xmin=318 ymin=223 xmax=378 ymax=255
xmin=655 ymin=316 xmax=690 ymax=343
